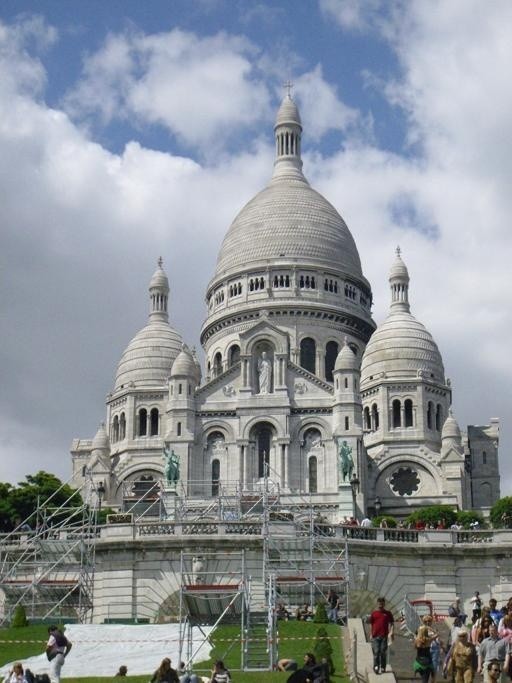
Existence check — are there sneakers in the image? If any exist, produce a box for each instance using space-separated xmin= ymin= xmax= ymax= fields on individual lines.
xmin=373 ymin=666 xmax=386 ymax=674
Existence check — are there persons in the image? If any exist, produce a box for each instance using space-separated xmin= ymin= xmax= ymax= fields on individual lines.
xmin=367 ymin=598 xmax=394 ymax=674
xmin=115 ymin=665 xmax=128 ymax=677
xmin=162 ymin=446 xmax=179 ymax=484
xmin=211 ymin=659 xmax=231 ymax=682
xmin=276 ymin=602 xmax=289 ymax=620
xmin=302 ymin=652 xmax=319 ymax=682
xmin=46 ymin=624 xmax=73 ymax=682
xmin=339 ymin=440 xmax=354 ymax=482
xmin=327 ymin=589 xmax=340 ymax=623
xmin=178 ymin=672 xmax=200 ymax=683
xmin=297 ymin=603 xmax=315 ymax=621
xmin=312 ymin=509 xmax=512 ymax=543
xmin=2 ymin=661 xmax=29 ymax=683
xmin=273 ymin=658 xmax=299 ymax=672
xmin=414 ymin=590 xmax=511 ymax=682
xmin=257 ymin=351 xmax=272 ymax=393
xmin=1 ymin=515 xmax=48 ymax=541
xmin=151 ymin=657 xmax=181 ymax=683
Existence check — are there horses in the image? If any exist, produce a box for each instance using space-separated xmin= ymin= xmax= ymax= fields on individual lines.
xmin=338 ymin=445 xmax=355 ymax=481
xmin=165 ymin=456 xmax=180 ymax=487
